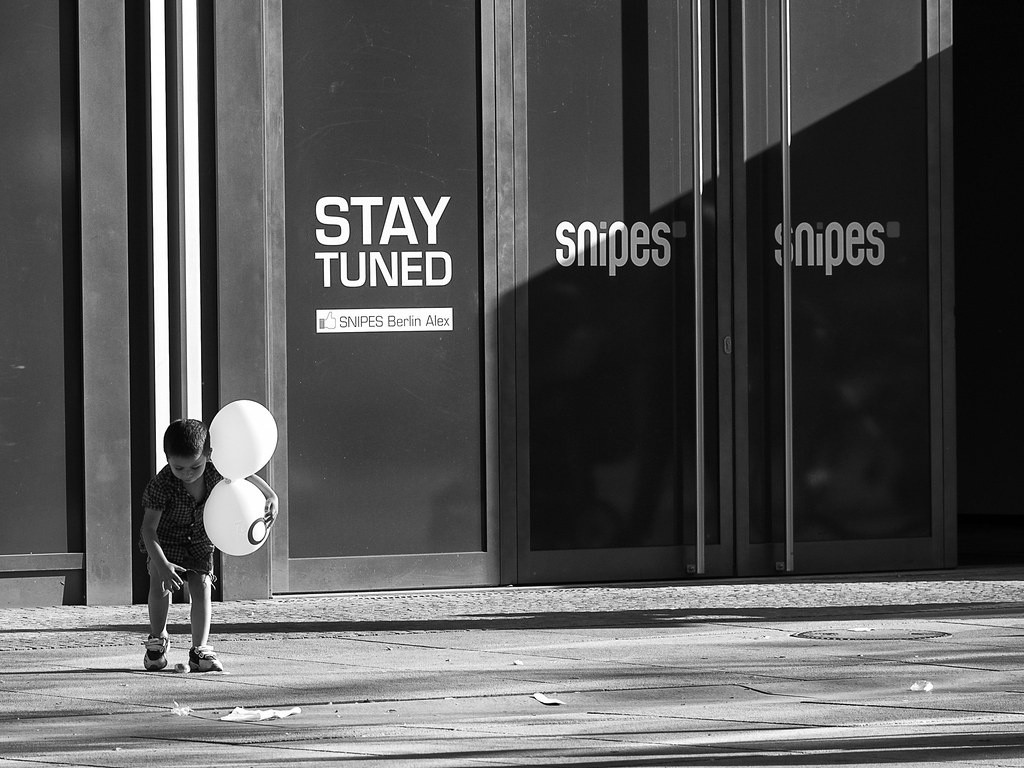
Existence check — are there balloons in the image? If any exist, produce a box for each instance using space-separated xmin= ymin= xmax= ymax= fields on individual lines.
xmin=208 ymin=399 xmax=279 ymax=480
xmin=203 ymin=479 xmax=270 ymax=557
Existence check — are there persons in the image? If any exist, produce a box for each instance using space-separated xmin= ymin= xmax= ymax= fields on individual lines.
xmin=138 ymin=419 xmax=279 ymax=671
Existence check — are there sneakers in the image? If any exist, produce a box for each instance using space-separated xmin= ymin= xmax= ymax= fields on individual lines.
xmin=188 ymin=645 xmax=223 ymax=673
xmin=143 ymin=633 xmax=171 ymax=670
